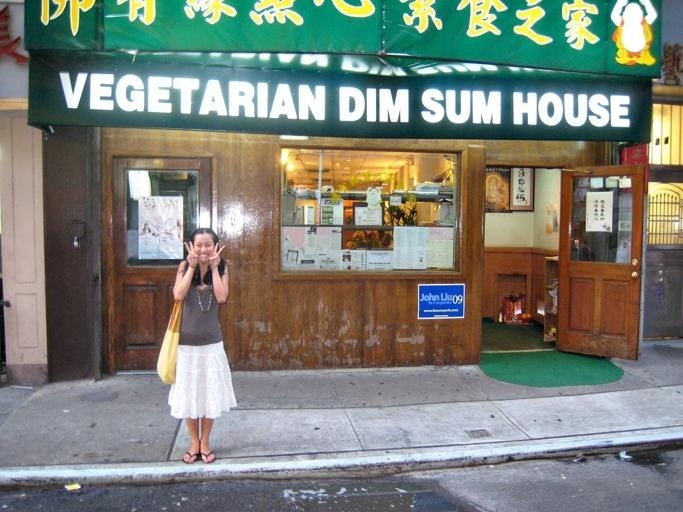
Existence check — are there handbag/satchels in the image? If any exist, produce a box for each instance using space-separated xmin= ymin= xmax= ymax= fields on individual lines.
xmin=156 ymin=299 xmax=182 ymax=385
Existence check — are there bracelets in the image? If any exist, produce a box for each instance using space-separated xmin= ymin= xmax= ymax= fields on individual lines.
xmin=188 ymin=265 xmax=196 ymax=269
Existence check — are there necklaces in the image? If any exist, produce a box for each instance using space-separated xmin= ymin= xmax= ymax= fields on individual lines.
xmin=195 ymin=282 xmax=213 ymax=312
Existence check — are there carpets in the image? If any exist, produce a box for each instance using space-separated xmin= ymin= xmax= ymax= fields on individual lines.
xmin=479 ymin=352 xmax=626 ymax=388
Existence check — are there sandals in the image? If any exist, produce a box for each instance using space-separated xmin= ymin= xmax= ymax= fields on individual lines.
xmin=199 ymin=442 xmax=215 ymax=463
xmin=183 ymin=441 xmax=199 ymax=464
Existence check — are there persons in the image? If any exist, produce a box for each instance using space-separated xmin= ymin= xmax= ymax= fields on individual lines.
xmin=156 ymin=228 xmax=239 ymax=464
xmin=140 ymin=221 xmax=152 ymax=237
